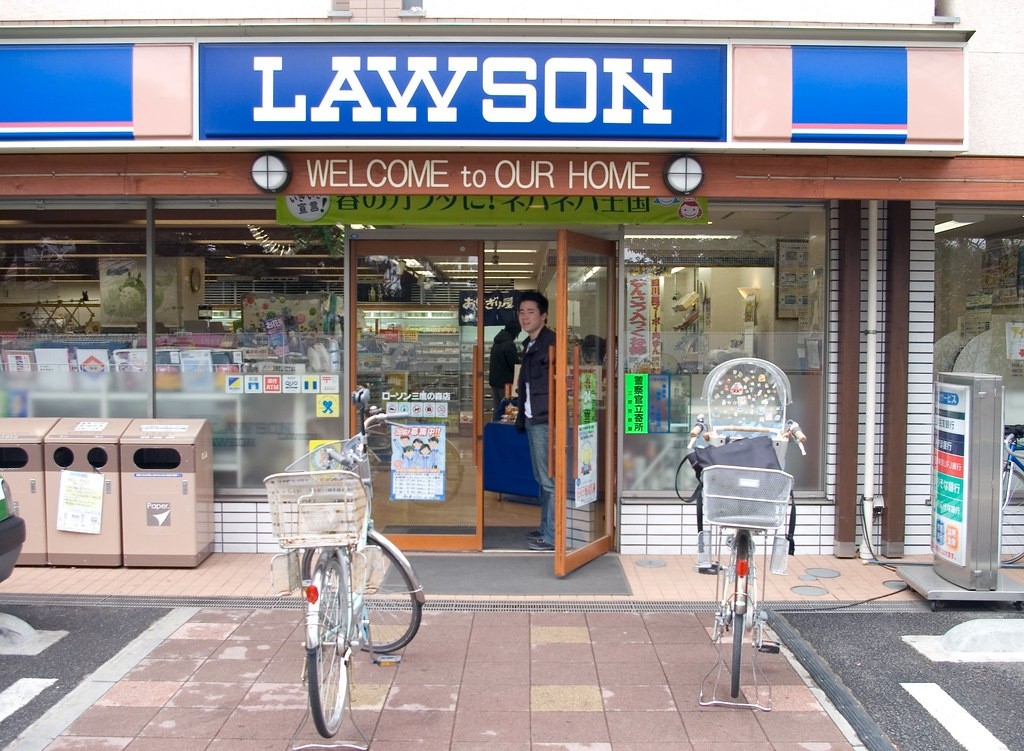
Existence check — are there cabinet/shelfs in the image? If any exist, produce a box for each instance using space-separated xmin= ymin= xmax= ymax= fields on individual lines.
xmin=0 ymin=308 xmax=619 ymax=498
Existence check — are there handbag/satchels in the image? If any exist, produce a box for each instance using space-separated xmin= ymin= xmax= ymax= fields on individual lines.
xmin=675 ymin=435 xmax=796 ymax=555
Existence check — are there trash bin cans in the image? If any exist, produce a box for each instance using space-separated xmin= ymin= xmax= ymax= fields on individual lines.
xmin=0 ymin=418 xmax=216 ymax=568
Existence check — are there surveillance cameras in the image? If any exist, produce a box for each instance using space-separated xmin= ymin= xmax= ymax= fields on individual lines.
xmin=492 ymin=255 xmax=500 ymax=265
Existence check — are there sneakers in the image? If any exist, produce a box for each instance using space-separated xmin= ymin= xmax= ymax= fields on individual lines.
xmin=526 ymin=529 xmax=544 ymax=539
xmin=528 ymin=539 xmax=555 ymax=550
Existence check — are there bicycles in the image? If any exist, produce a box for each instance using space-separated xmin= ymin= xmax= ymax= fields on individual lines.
xmin=686 ymin=413 xmax=807 ymax=712
xmin=1002 ymin=423 xmax=1024 ymax=566
xmin=264 ymin=406 xmax=425 ymax=751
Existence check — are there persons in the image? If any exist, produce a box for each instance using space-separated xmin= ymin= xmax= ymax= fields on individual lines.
xmin=514 ymin=288 xmax=558 ymax=552
xmin=490 ymin=315 xmax=522 ymax=422
xmin=582 ymin=335 xmax=619 ymax=379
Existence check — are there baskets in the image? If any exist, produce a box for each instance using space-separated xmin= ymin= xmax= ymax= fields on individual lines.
xmin=263 ymin=469 xmax=368 ymax=549
xmin=700 ymin=464 xmax=795 ymax=529
xmin=284 ymin=439 xmax=374 ymax=499
